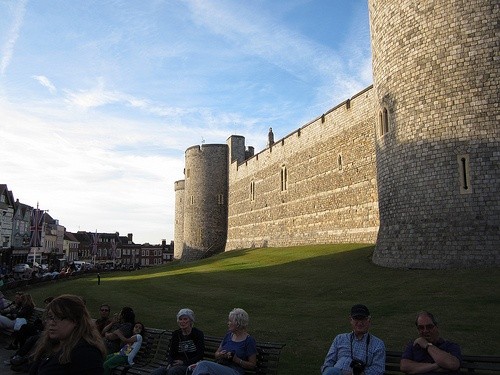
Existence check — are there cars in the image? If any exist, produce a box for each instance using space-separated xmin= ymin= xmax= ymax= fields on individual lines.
xmin=13 ymin=257 xmax=141 ymax=277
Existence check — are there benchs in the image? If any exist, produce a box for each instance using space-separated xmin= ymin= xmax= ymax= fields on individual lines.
xmin=0 ymin=307 xmax=45 ymax=347
xmin=118 ymin=327 xmax=166 ymax=375
xmin=386 ymin=349 xmax=500 ymax=375
xmin=0 ymin=268 xmax=107 ymax=292
xmin=138 ymin=330 xmax=286 ymax=375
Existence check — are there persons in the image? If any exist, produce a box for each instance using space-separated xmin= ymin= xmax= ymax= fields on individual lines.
xmin=151 ymin=308 xmax=204 ymax=375
xmin=186 ymin=307 xmax=258 ymax=375
xmin=92 ymin=303 xmax=112 ymax=334
xmin=103 ymin=321 xmax=145 ymax=375
xmin=320 ymin=303 xmax=387 ymax=375
xmin=102 ymin=305 xmax=136 ymax=355
xmin=30 ymin=292 xmax=107 ymax=375
xmin=1 ymin=291 xmax=53 ymax=370
xmin=96 ymin=273 xmax=101 ymax=285
xmin=400 ymin=309 xmax=462 ymax=375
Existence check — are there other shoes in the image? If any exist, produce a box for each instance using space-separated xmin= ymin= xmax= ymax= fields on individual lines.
xmin=2 ymin=339 xmax=33 ymax=373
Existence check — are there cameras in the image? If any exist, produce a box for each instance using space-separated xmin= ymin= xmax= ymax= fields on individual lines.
xmin=350 ymin=359 xmax=365 ymax=375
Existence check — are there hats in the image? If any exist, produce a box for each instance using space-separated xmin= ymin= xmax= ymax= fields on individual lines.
xmin=350 ymin=304 xmax=370 ymax=320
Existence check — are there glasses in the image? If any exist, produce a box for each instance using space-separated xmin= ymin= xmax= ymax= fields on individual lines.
xmin=100 ymin=309 xmax=110 ymax=312
xmin=418 ymin=324 xmax=437 ymax=329
xmin=43 ymin=316 xmax=67 ymax=322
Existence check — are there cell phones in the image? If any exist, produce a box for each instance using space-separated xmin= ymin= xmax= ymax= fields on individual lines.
xmin=166 ymin=355 xmax=179 ymax=366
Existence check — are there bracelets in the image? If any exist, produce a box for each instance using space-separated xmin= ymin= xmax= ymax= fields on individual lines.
xmin=239 ymin=359 xmax=243 ymax=366
xmin=426 ymin=342 xmax=433 ymax=350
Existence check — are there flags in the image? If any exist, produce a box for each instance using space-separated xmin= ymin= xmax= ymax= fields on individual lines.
xmin=110 ymin=242 xmax=117 ymax=257
xmin=90 ymin=233 xmax=100 ymax=255
xmin=29 ymin=209 xmax=44 ymax=247
